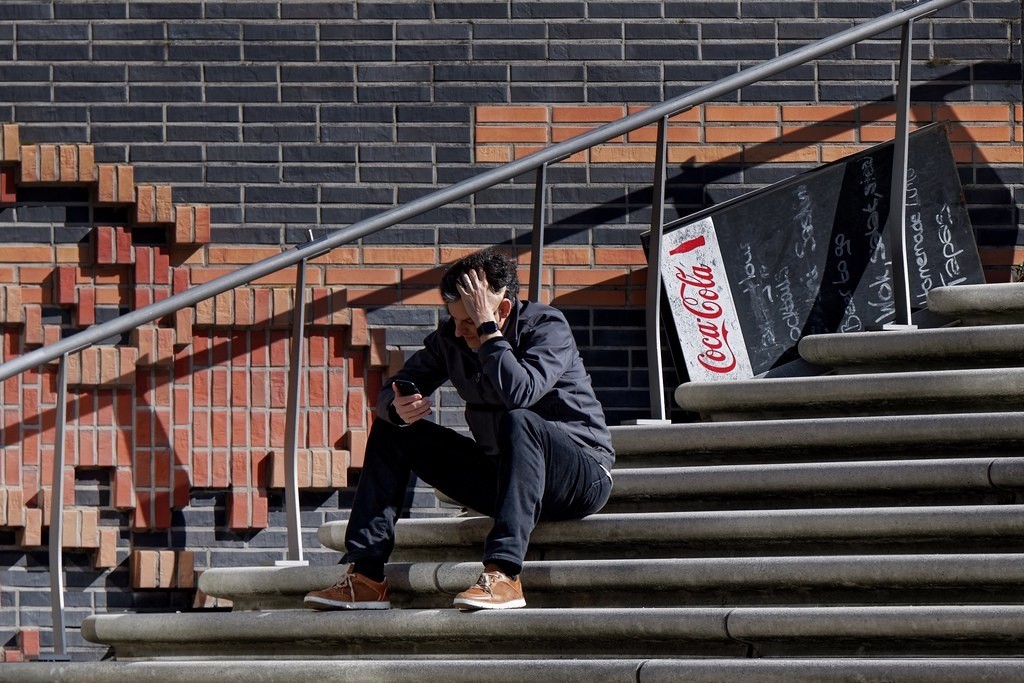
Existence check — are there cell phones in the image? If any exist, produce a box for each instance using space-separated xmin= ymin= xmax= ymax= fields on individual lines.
xmin=395 ymin=380 xmax=433 ymax=415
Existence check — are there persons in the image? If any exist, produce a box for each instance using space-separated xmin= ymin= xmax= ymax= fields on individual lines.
xmin=304 ymin=247 xmax=617 ymax=611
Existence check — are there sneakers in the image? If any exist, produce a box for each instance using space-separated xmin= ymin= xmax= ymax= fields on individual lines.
xmin=453 ymin=568 xmax=527 ymax=608
xmin=304 ymin=565 xmax=391 ymax=611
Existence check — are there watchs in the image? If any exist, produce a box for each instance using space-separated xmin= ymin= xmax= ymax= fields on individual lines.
xmin=476 ymin=321 xmax=500 ymax=338
